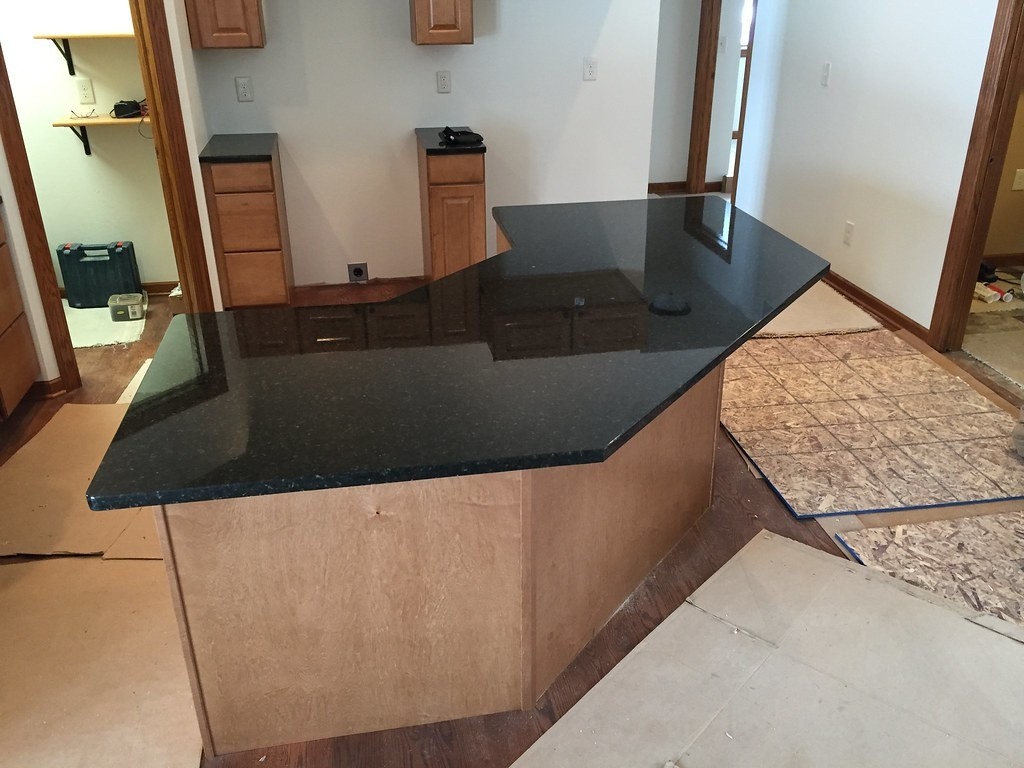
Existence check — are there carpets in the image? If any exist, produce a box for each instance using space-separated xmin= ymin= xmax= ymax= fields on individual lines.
xmin=61 ymin=298 xmax=151 ymax=353
xmin=752 ymin=279 xmax=882 ymax=338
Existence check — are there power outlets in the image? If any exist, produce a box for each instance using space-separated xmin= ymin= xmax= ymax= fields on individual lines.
xmin=76 ymin=78 xmax=95 ymax=103
xmin=436 ymin=70 xmax=451 ymax=93
xmin=234 ymin=76 xmax=254 ymax=102
xmin=844 ymin=221 xmax=854 ymax=247
xmin=583 ymin=58 xmax=597 ymax=80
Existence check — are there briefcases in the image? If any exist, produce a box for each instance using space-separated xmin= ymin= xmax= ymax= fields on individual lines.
xmin=56 ymin=241 xmax=142 ymax=308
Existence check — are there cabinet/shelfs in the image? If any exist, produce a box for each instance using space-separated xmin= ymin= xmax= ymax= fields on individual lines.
xmin=409 ymin=0 xmax=474 ymax=46
xmin=198 ymin=132 xmax=295 ymax=309
xmin=33 ymin=34 xmax=151 ymax=156
xmin=184 ymin=0 xmax=267 ymax=51
xmin=416 ymin=126 xmax=486 ymax=283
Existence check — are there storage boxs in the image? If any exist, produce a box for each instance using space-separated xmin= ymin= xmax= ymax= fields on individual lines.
xmin=108 ymin=294 xmax=143 ymax=321
